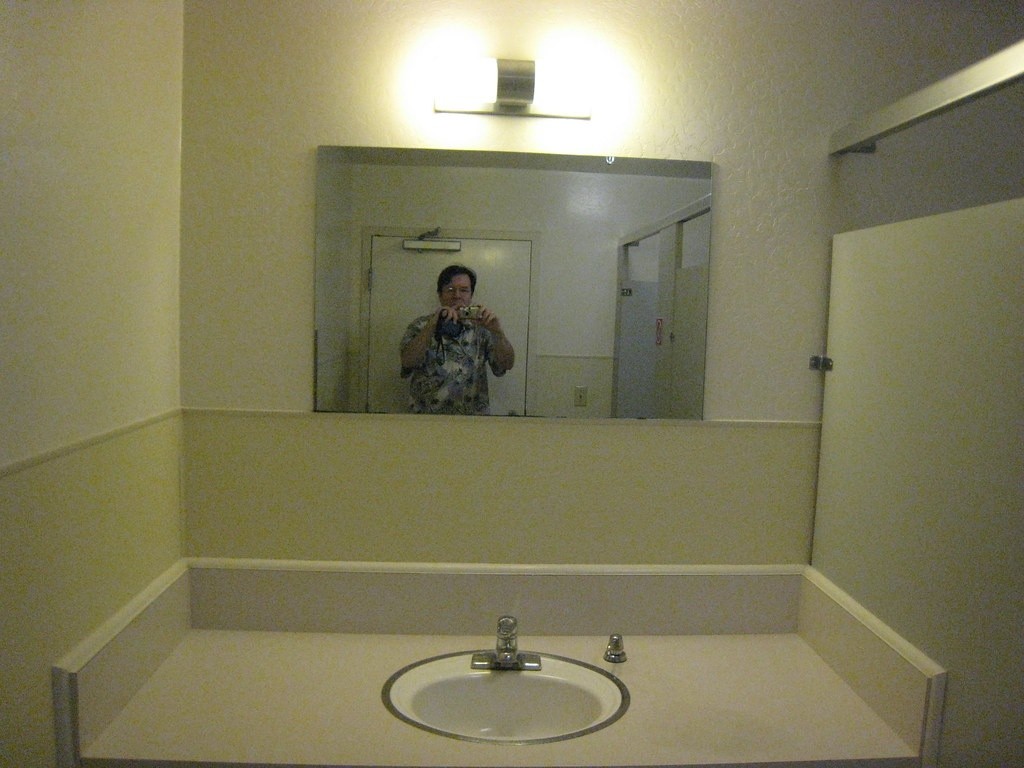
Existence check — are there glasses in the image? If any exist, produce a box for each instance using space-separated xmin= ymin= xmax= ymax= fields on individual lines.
xmin=441 ymin=286 xmax=472 ymax=296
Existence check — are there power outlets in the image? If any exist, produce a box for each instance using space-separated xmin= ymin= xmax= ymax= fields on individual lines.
xmin=573 ymin=386 xmax=587 ymax=406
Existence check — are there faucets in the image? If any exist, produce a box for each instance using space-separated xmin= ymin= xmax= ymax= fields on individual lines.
xmin=470 ymin=617 xmax=542 ymax=673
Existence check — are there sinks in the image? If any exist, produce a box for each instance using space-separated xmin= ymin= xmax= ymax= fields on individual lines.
xmin=412 ymin=672 xmax=603 ymax=740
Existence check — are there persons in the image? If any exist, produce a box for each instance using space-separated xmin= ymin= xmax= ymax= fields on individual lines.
xmin=399 ymin=265 xmax=515 ymax=416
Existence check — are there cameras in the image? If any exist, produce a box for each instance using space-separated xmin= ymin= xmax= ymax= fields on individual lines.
xmin=460 ymin=307 xmax=479 ymax=319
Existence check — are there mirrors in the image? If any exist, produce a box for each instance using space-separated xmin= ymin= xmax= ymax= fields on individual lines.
xmin=435 ymin=57 xmax=592 ymax=120
xmin=313 ymin=145 xmax=711 ymax=420
